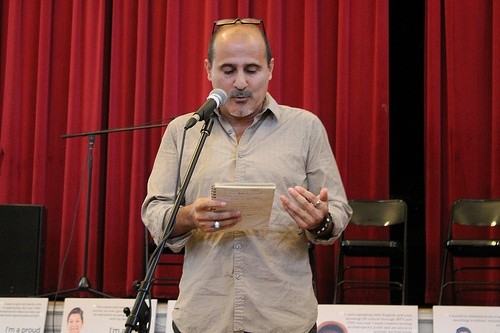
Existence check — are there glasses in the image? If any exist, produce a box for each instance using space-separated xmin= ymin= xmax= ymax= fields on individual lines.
xmin=211 ymin=18 xmax=267 ymax=39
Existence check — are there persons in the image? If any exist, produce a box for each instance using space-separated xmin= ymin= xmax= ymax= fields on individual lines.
xmin=141 ymin=18 xmax=353 ymax=333
xmin=67 ymin=308 xmax=83 ymax=333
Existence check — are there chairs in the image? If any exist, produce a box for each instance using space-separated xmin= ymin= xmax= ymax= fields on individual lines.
xmin=438 ymin=198 xmax=500 ymax=306
xmin=331 ymin=199 xmax=409 ymax=305
xmin=145 ymin=225 xmax=186 ymax=299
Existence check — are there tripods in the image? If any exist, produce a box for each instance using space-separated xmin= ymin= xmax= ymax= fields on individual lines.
xmin=40 ymin=123 xmax=171 ymax=299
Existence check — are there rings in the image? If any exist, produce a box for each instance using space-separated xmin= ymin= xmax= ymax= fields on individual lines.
xmin=316 ymin=200 xmax=321 ymax=206
xmin=214 ymin=221 xmax=220 ymax=229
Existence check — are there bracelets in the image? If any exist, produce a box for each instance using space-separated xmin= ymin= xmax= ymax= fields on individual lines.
xmin=307 ymin=214 xmax=330 ymax=235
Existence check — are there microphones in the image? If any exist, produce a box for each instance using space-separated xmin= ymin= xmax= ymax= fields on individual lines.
xmin=183 ymin=88 xmax=227 ymax=130
